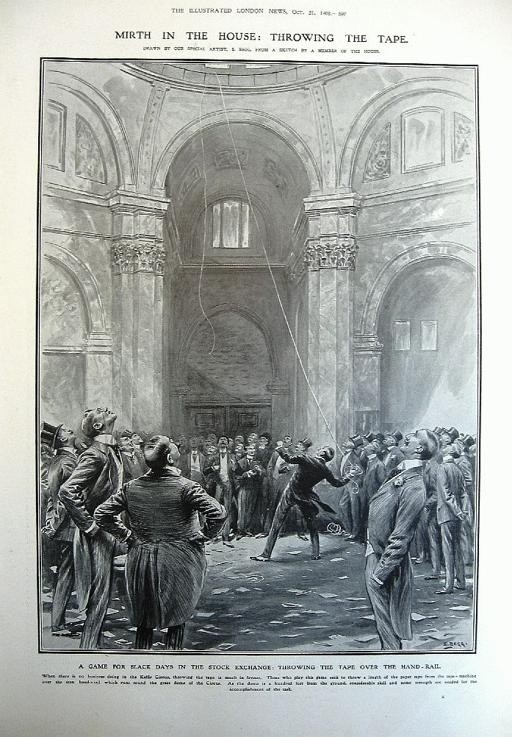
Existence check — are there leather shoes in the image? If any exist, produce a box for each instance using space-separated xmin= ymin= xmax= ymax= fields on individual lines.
xmin=51 ymin=629 xmax=83 ymax=641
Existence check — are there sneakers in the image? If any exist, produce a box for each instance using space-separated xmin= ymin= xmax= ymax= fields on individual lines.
xmin=222 ymin=531 xmax=322 ymax=562
xmin=412 ymin=555 xmax=466 ymax=595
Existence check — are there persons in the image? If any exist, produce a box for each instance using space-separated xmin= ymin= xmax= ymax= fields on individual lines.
xmin=41 ymin=403 xmax=476 ymax=652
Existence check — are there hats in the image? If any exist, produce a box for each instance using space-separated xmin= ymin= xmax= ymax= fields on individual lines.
xmin=118 ymin=426 xmax=476 ymax=452
xmin=40 ymin=420 xmax=64 ymax=452
